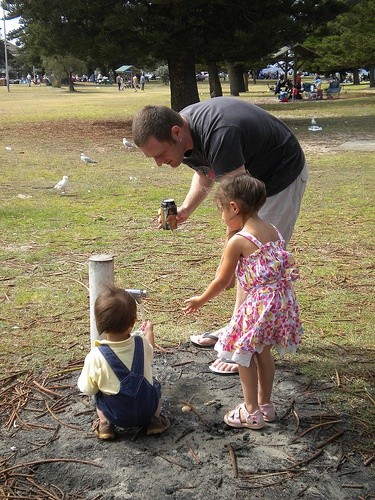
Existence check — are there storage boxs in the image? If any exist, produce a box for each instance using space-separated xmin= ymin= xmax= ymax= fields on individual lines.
xmin=280 ymin=93 xmax=287 ymax=100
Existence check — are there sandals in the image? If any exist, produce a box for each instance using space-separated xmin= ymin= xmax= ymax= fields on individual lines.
xmin=222 ymin=402 xmax=265 ymax=430
xmin=259 ymin=402 xmax=276 ymax=422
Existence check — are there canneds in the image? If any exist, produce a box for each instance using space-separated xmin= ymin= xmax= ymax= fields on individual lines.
xmin=161 ymin=199 xmax=177 ymax=230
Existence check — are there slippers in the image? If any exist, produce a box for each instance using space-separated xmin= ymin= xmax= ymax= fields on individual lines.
xmin=189 ymin=332 xmax=218 ymax=347
xmin=208 ymin=358 xmax=239 ymax=375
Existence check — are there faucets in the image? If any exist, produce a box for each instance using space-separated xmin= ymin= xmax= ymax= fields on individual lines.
xmin=124 ymin=289 xmax=142 ymax=305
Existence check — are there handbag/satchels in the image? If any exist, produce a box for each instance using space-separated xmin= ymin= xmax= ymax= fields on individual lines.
xmin=274 ymin=85 xmax=279 ymax=95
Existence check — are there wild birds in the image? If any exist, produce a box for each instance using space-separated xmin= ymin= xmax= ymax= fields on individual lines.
xmin=121 ymin=136 xmax=136 ymax=152
xmin=50 ymin=173 xmax=72 ymax=193
xmin=310 ymin=114 xmax=319 ymax=128
xmin=80 ymin=152 xmax=99 ymax=167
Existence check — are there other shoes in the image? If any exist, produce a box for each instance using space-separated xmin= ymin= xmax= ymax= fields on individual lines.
xmin=91 ymin=417 xmax=115 ymax=440
xmin=146 ymin=411 xmax=170 ymax=434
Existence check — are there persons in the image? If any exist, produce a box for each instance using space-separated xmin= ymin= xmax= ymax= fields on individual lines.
xmin=274 ymin=71 xmax=323 ymax=101
xmin=77 ymin=282 xmax=169 ymax=439
xmin=133 ymin=73 xmax=145 ymax=92
xmin=27 ymin=73 xmax=32 ymax=87
xmin=132 ymin=95 xmax=308 ymax=375
xmin=183 ymin=170 xmax=304 ymax=431
xmin=116 ymin=73 xmax=124 ymax=90
